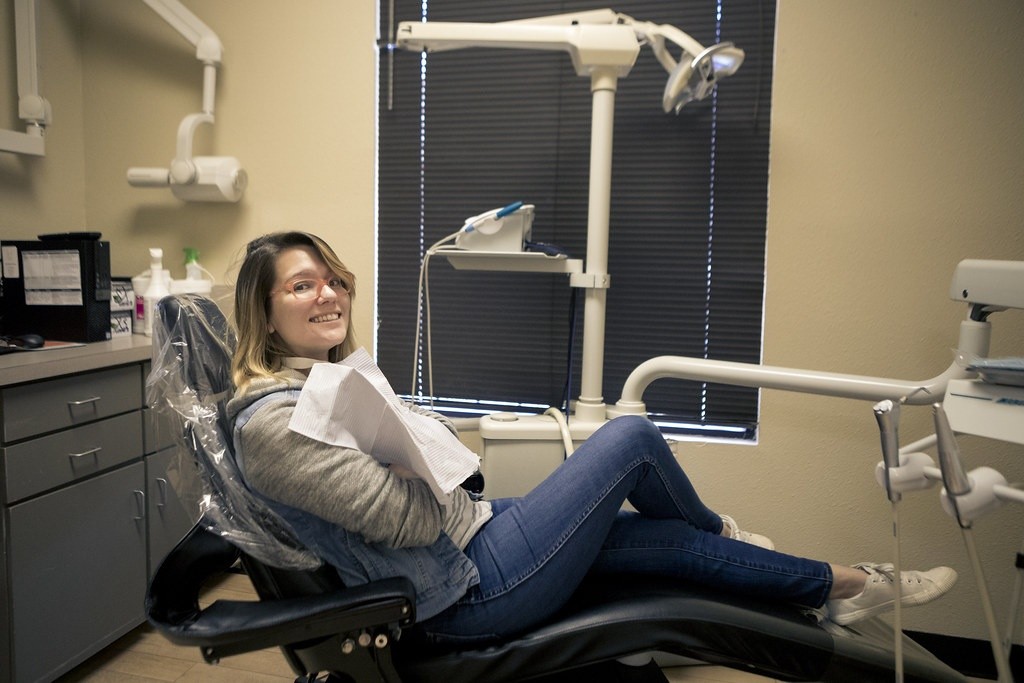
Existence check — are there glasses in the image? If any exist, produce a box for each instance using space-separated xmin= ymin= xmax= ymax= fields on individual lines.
xmin=266 ymin=272 xmax=355 ymax=301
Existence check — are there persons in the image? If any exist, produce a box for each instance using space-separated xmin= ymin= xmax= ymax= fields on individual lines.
xmin=227 ymin=232 xmax=959 ymax=641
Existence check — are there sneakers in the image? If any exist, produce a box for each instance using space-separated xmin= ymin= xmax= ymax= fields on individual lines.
xmin=829 ymin=555 xmax=958 ymax=625
xmin=717 ymin=513 xmax=774 ymax=550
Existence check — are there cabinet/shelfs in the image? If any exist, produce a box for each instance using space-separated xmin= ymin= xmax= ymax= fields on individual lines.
xmin=0 ymin=359 xmax=204 ymax=683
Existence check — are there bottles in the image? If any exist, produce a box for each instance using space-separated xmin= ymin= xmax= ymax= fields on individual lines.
xmin=144 ymin=248 xmax=170 ymax=337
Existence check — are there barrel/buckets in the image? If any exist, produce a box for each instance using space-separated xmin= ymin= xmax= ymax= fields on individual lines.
xmin=170 ymin=279 xmax=210 ymax=298
xmin=132 ymin=269 xmax=170 ymax=334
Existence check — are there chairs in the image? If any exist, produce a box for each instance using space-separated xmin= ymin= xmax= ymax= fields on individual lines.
xmin=143 ymin=293 xmax=968 ymax=683
